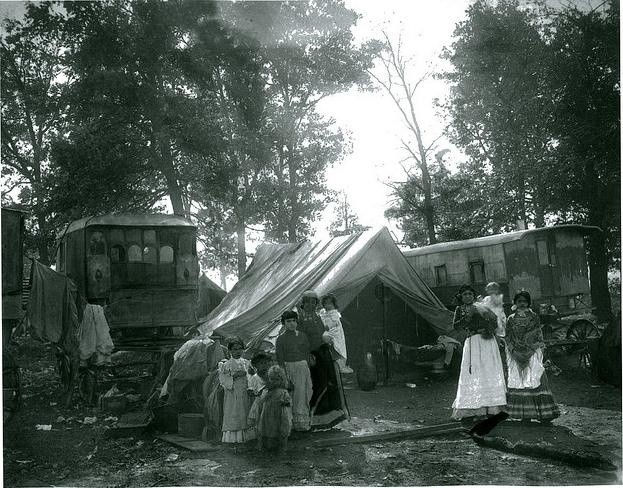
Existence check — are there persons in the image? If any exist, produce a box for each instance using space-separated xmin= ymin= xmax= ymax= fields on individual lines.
xmin=450 ymin=284 xmax=507 ymax=422
xmin=218 ymin=290 xmax=350 ymax=452
xmin=502 ymin=291 xmax=562 ymax=426
xmin=477 ymin=281 xmax=507 ymax=347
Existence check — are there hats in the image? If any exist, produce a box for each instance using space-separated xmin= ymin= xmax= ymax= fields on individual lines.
xmin=302 ymin=290 xmax=320 ymax=304
